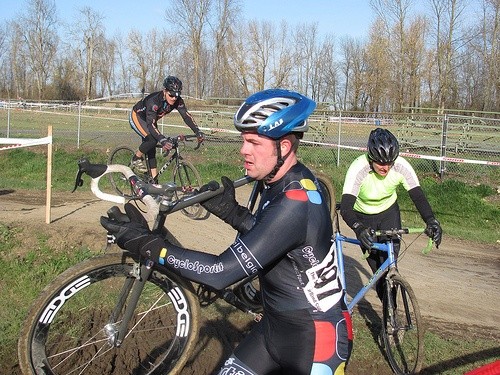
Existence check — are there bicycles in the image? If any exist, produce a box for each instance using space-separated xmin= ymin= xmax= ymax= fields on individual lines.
xmin=329 ymin=201 xmax=435 ymax=375
xmin=17 ymin=157 xmax=335 ymax=375
xmin=107 ymin=130 xmax=205 ymax=220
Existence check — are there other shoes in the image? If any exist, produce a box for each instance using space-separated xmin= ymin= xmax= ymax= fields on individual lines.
xmin=128 ymin=158 xmax=149 ymax=175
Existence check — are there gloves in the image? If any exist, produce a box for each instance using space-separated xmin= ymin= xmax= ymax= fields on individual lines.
xmin=195 ymin=130 xmax=205 ymax=142
xmin=158 ymin=135 xmax=173 ymax=151
xmin=424 ymin=217 xmax=443 ymax=246
xmin=193 ymin=174 xmax=251 ymax=230
xmin=356 ymin=227 xmax=375 ymax=250
xmin=99 ymin=202 xmax=164 ymax=268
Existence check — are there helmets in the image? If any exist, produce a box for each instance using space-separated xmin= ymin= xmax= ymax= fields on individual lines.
xmin=162 ymin=74 xmax=183 ymax=97
xmin=366 ymin=126 xmax=402 ymax=165
xmin=232 ymin=87 xmax=318 ymax=141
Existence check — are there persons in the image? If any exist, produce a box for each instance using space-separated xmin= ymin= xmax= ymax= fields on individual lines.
xmin=128 ymin=76 xmax=202 ymax=185
xmin=101 ymin=90 xmax=353 ymax=375
xmin=339 ymin=128 xmax=444 ymax=349
